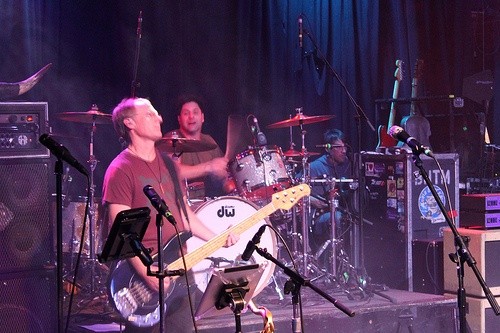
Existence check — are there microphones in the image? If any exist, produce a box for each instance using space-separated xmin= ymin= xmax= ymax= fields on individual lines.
xmin=253 ymin=116 xmax=267 ymax=146
xmin=242 ymin=224 xmax=265 ymax=261
xmin=41 ymin=133 xmax=89 ymax=175
xmin=390 ymin=125 xmax=434 ymax=157
xmin=143 ymin=185 xmax=176 ymax=224
xmin=298 ymin=17 xmax=303 ymax=48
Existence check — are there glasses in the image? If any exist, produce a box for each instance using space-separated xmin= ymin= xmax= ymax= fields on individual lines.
xmin=129 ymin=113 xmax=162 ymax=120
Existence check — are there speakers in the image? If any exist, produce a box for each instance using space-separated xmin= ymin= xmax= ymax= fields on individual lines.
xmin=0 ymin=272 xmax=59 ymax=333
xmin=442 ymin=226 xmax=500 ymax=297
xmin=0 ymin=158 xmax=58 ymax=272
xmin=62 ymin=196 xmax=108 ymax=256
xmin=443 ymin=293 xmax=500 ymax=333
xmin=356 ymin=152 xmax=458 ymax=293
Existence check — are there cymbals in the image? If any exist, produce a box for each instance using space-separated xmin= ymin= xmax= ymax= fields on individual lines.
xmin=263 ymin=113 xmax=338 ymax=129
xmin=53 ymin=110 xmax=114 ymax=125
xmin=283 ymin=149 xmax=321 ymax=157
xmin=154 ymin=137 xmax=217 ymax=154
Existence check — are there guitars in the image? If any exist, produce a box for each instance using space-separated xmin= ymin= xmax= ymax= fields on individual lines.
xmin=399 ymin=57 xmax=433 ymax=153
xmin=107 ymin=177 xmax=312 ymax=330
xmin=376 ymin=59 xmax=404 ymax=150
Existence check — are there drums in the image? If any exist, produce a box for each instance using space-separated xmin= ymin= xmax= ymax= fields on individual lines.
xmin=51 ymin=194 xmax=103 ymax=259
xmin=182 ymin=196 xmax=277 ymax=308
xmin=226 ymin=145 xmax=291 ymax=200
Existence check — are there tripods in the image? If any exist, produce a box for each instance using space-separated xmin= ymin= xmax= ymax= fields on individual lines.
xmin=272 ymin=126 xmax=395 ymax=305
xmin=58 ymin=118 xmax=116 ymax=316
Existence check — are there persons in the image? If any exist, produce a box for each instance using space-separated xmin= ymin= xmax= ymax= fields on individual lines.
xmin=102 ymin=97 xmax=237 ymax=333
xmin=161 ymin=96 xmax=237 ymax=213
xmin=294 ymin=128 xmax=370 ymax=239
